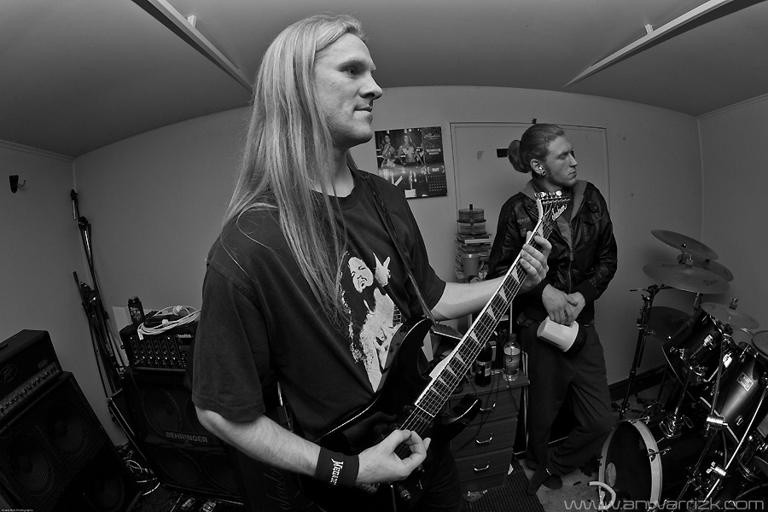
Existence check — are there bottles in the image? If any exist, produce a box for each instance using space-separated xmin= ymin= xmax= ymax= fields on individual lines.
xmin=502 ymin=334 xmax=521 ymax=382
xmin=127 ymin=296 xmax=144 ymax=323
xmin=475 ymin=342 xmax=493 ymax=387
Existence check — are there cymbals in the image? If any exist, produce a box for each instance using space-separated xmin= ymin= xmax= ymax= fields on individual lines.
xmin=700 ymin=302 xmax=758 ymax=329
xmin=751 ymin=330 xmax=768 ymax=355
xmin=643 ymin=261 xmax=731 ymax=294
xmin=651 ymin=228 xmax=718 ymax=259
xmin=643 ymin=307 xmax=689 ymax=347
xmin=677 ymin=253 xmax=733 ymax=281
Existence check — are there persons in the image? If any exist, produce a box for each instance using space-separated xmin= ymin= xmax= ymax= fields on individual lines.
xmin=340 ymin=250 xmax=411 ymax=396
xmin=375 ymin=134 xmax=428 ymax=197
xmin=487 ymin=124 xmax=618 ymax=490
xmin=190 ymin=13 xmax=552 ymax=511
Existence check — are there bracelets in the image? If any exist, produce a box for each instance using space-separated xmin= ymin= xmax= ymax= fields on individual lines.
xmin=313 ymin=445 xmax=359 ymax=490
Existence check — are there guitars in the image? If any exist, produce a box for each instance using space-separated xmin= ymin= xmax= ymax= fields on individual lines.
xmin=315 ymin=191 xmax=570 ymax=510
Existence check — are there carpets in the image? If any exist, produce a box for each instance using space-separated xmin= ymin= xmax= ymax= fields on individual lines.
xmin=457 ymin=461 xmax=546 ymax=512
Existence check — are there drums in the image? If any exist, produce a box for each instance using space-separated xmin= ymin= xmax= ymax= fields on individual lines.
xmin=597 ymin=412 xmax=726 ymax=511
xmin=661 ymin=313 xmax=737 ymax=401
xmin=698 ymin=343 xmax=768 ymax=453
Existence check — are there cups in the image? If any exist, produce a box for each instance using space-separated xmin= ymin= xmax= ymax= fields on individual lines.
xmin=460 ymin=253 xmax=480 ymax=277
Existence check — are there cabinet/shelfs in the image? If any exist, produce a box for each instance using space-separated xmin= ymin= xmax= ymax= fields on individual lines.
xmin=440 ymin=370 xmax=530 ymax=493
